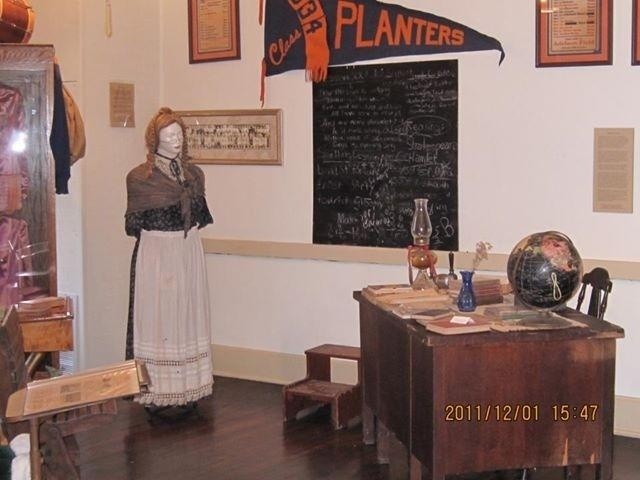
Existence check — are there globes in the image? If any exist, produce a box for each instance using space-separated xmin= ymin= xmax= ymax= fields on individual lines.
xmin=471 ymin=231 xmax=584 ymax=312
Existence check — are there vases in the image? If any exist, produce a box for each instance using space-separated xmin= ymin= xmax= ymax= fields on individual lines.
xmin=457 ymin=271 xmax=476 ymax=312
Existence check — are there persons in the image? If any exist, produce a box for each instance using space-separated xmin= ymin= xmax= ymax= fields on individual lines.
xmin=122 ymin=107 xmax=214 ymax=433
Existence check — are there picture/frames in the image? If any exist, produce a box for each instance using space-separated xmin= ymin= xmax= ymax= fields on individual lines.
xmin=536 ymin=0 xmax=613 ymax=66
xmin=188 ymin=0 xmax=241 ymax=64
xmin=174 ymin=109 xmax=283 ymax=165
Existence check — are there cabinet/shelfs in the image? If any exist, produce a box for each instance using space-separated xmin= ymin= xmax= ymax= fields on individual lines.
xmin=0 ymin=41 xmax=59 ymax=367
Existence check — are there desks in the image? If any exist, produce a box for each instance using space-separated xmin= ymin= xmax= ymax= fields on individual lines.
xmin=353 ymin=289 xmax=625 ymax=480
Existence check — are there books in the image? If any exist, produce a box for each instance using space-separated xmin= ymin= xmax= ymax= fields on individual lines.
xmin=361 ymin=276 xmax=513 ymax=336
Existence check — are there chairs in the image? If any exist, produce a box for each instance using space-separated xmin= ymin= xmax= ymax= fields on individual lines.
xmin=577 ymin=267 xmax=612 ymax=320
xmin=282 ymin=344 xmax=362 ymax=431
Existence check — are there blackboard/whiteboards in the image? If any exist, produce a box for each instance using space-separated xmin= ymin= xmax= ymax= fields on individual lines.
xmin=312 ymin=60 xmax=459 ymax=251
